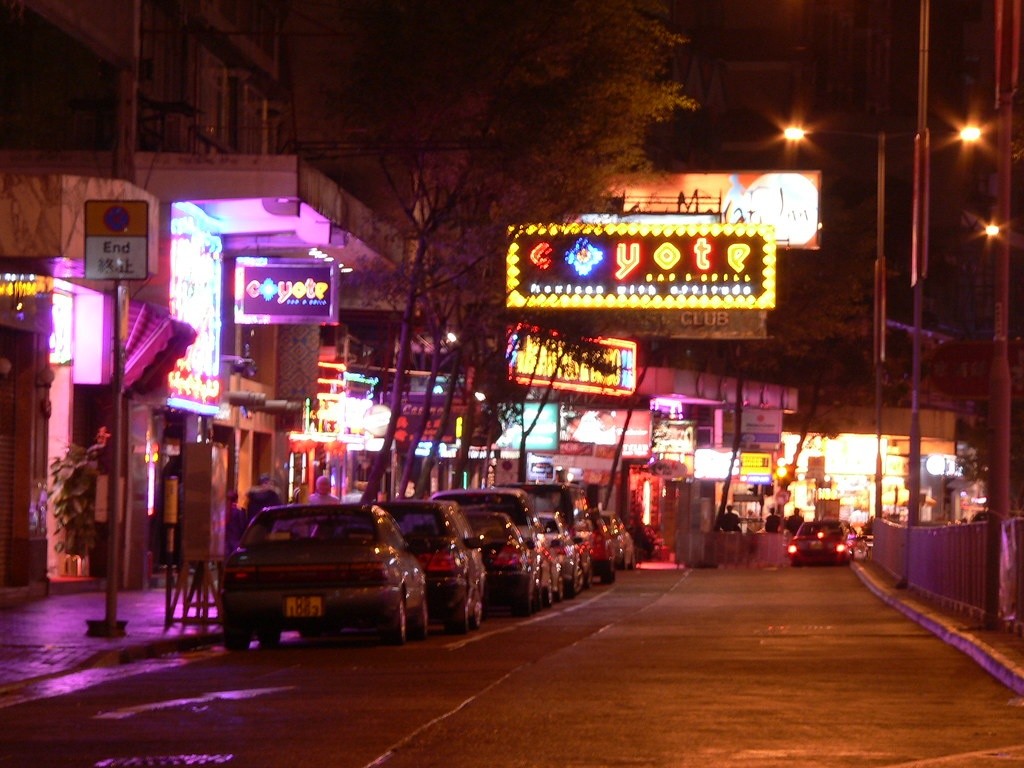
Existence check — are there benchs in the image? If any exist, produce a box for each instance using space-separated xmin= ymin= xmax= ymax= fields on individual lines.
xmin=271 ymin=527 xmax=374 ymax=542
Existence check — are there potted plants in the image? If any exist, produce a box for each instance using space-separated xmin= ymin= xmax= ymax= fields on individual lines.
xmin=48 ymin=441 xmax=98 ymax=578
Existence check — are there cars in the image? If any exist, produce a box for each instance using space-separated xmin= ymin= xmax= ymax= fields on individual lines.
xmin=788 ymin=521 xmax=859 ymax=567
xmin=217 ymin=500 xmax=430 ymax=651
xmin=429 ymin=480 xmax=637 ymax=620
xmin=344 ymin=497 xmax=487 ymax=637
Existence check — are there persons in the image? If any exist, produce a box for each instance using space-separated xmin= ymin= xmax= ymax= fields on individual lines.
xmin=244 ymin=475 xmax=286 ymax=529
xmin=785 ymin=508 xmax=804 ymax=536
xmin=719 ymin=505 xmax=742 ymax=533
xmin=303 ymin=475 xmax=341 ymax=504
xmin=226 ymin=490 xmax=247 ymax=560
xmin=764 ymin=507 xmax=781 ymax=533
xmin=745 ymin=510 xmax=764 ymax=532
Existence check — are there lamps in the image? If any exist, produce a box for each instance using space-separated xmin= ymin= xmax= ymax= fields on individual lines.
xmin=220 ymin=355 xmax=259 ymax=381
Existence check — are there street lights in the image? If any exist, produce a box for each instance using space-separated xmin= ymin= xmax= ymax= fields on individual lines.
xmin=781 ymin=124 xmax=884 ymax=519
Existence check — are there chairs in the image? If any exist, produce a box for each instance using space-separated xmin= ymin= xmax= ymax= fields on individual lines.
xmin=413 ymin=522 xmax=437 ymax=540
